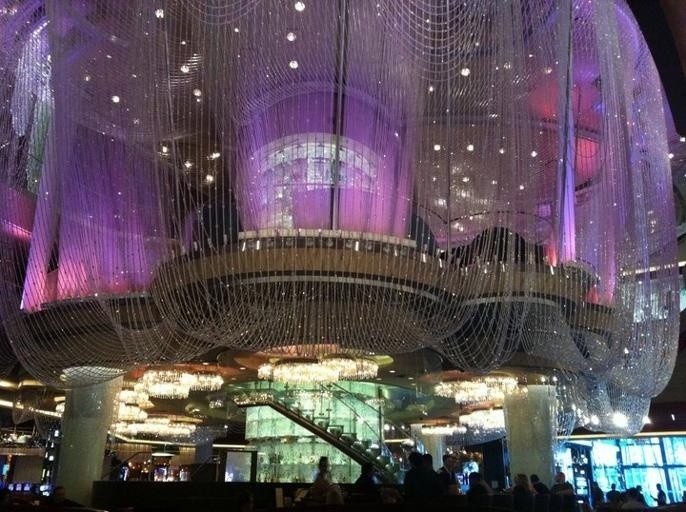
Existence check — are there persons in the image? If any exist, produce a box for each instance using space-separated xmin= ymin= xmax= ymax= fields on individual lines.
xmin=404 ymin=452 xmax=460 ymax=495
xmin=591 ymin=482 xmax=604 ymax=512
xmin=462 ymin=456 xmax=483 ymax=478
xmin=512 ymin=472 xmax=574 ymax=512
xmin=45 ymin=487 xmax=86 ymax=512
xmin=606 ymin=484 xmax=649 ymax=512
xmin=654 ymin=484 xmax=666 ymax=506
xmin=353 ymin=463 xmax=375 ymax=484
xmin=467 ymin=472 xmax=494 ymax=496
xmin=311 ymin=456 xmax=335 ymax=512
